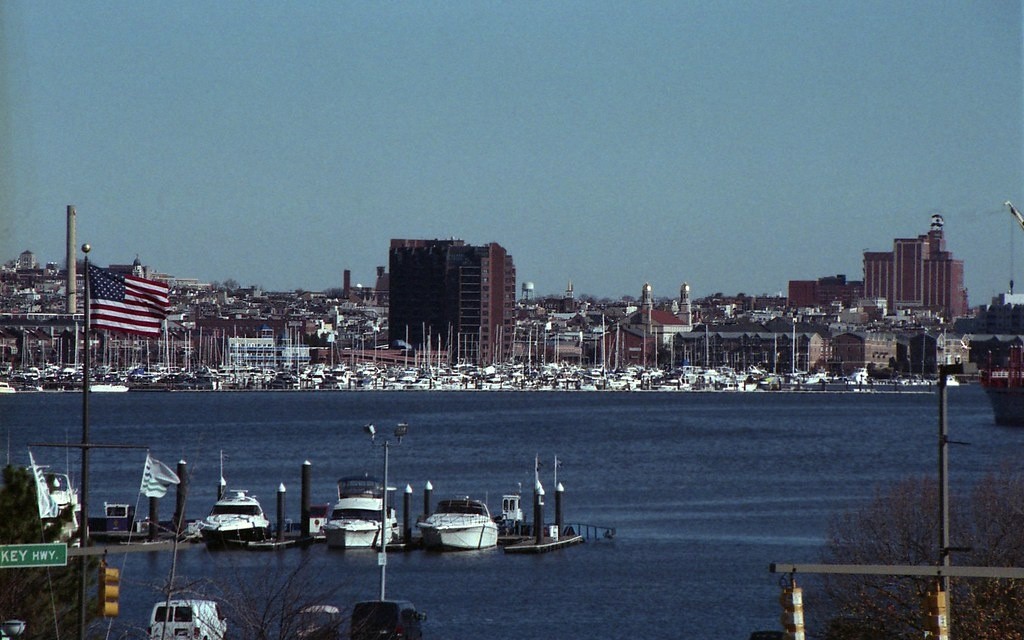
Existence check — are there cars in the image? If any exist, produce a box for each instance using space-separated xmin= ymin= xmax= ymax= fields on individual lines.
xmin=0 ymin=366 xmax=221 ymax=381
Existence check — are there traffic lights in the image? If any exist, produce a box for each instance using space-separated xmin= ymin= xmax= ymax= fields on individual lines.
xmin=97 ymin=561 xmax=122 ymax=622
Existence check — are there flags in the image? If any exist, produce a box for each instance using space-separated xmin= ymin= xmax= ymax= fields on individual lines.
xmin=89 ymin=264 xmax=172 ymax=336
xmin=141 ymin=457 xmax=180 ymax=498
xmin=30 ymin=451 xmax=61 ymax=517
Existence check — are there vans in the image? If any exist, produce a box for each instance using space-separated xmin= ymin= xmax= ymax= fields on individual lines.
xmin=351 ymin=599 xmax=427 ymax=640
xmin=145 ymin=600 xmax=228 ymax=640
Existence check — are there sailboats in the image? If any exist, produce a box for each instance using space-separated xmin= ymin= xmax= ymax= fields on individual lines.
xmin=141 ymin=329 xmax=924 ymax=391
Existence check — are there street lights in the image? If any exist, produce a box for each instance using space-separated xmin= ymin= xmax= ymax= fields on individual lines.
xmin=364 ymin=421 xmax=410 ymax=600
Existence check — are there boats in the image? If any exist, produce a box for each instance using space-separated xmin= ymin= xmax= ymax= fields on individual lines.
xmin=980 ymin=345 xmax=1024 ymax=423
xmin=201 ymin=489 xmax=271 ymax=542
xmin=38 ymin=473 xmax=78 ymax=516
xmin=323 ymin=473 xmax=400 ymax=547
xmin=415 ymin=481 xmax=497 ymax=553
xmin=0 ymin=382 xmax=18 ymax=394
xmin=88 ymin=383 xmax=129 ymax=393
